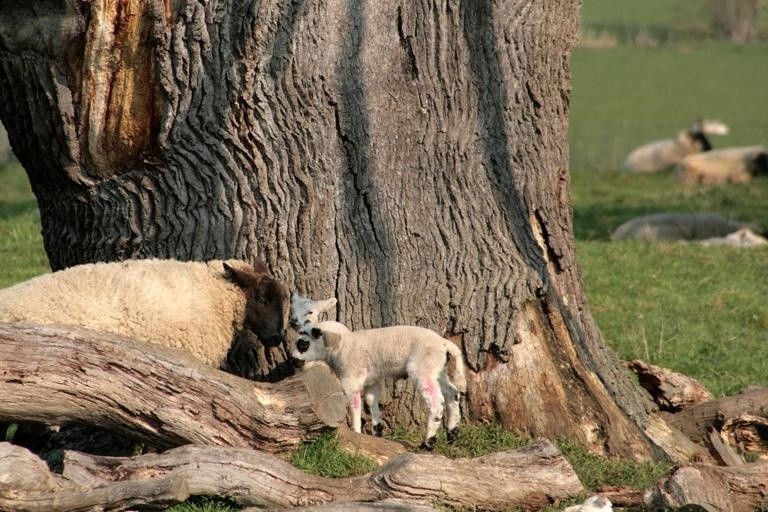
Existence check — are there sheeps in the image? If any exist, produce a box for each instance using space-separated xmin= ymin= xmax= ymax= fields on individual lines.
xmin=623 ymin=128 xmax=712 ymax=174
xmin=0 ymin=256 xmax=292 ymax=370
xmin=290 ymin=320 xmax=467 ymax=452
xmin=681 ymin=145 xmax=768 ymax=187
xmin=610 ymin=211 xmax=768 ymax=249
xmin=694 ymin=115 xmax=728 ymax=136
xmin=290 ymin=293 xmax=338 ymax=330
